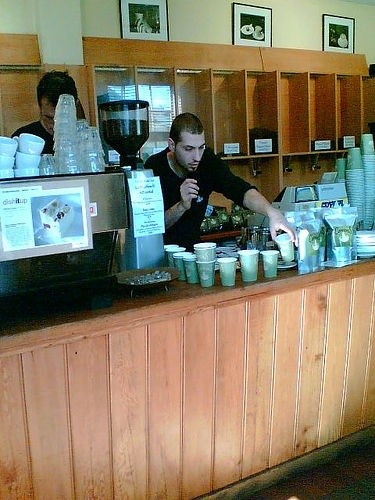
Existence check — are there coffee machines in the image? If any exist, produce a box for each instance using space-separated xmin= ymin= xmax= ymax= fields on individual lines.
xmin=100 ymin=99 xmax=167 ymax=270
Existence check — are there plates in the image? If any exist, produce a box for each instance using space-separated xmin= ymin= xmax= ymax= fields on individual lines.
xmin=241 ymin=25 xmax=264 ymax=40
xmin=338 ymin=38 xmax=347 ymax=47
xmin=355 ymin=231 xmax=375 ymax=258
xmin=277 ymin=261 xmax=297 ymax=269
xmin=116 ymin=269 xmax=181 ymax=289
xmin=214 ymin=246 xmax=241 ymax=270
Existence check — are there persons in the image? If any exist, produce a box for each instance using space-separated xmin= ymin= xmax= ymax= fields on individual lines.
xmin=10 ymin=71 xmax=78 ymax=156
xmin=359 ymin=122 xmax=375 ymax=149
xmin=145 ymin=112 xmax=296 ymax=254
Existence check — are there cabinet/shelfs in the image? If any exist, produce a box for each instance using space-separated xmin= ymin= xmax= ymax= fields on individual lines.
xmin=0 ymin=33 xmax=375 ymax=241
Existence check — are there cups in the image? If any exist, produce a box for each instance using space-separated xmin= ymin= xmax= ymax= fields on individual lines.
xmin=260 ymin=250 xmax=280 ymax=277
xmin=183 ymin=255 xmax=199 ymax=284
xmin=39 ymin=95 xmax=106 ymax=176
xmin=236 ymin=250 xmax=260 ymax=282
xmin=340 ymin=34 xmax=346 ymax=45
xmin=255 ymin=26 xmax=263 ymax=37
xmin=194 ymin=243 xmax=217 ymax=288
xmin=0 ymin=133 xmax=46 ymax=178
xmin=164 ymin=244 xmax=193 ymax=281
xmin=240 ymin=225 xmax=276 ymax=251
xmin=276 ymin=233 xmax=295 ymax=262
xmin=334 ymin=134 xmax=375 ymax=230
xmin=217 ymin=257 xmax=238 ymax=286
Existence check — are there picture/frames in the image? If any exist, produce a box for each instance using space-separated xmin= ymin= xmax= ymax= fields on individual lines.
xmin=232 ymin=2 xmax=273 ymax=47
xmin=321 ymin=14 xmax=355 ymax=54
xmin=118 ymin=0 xmax=170 ymax=41
